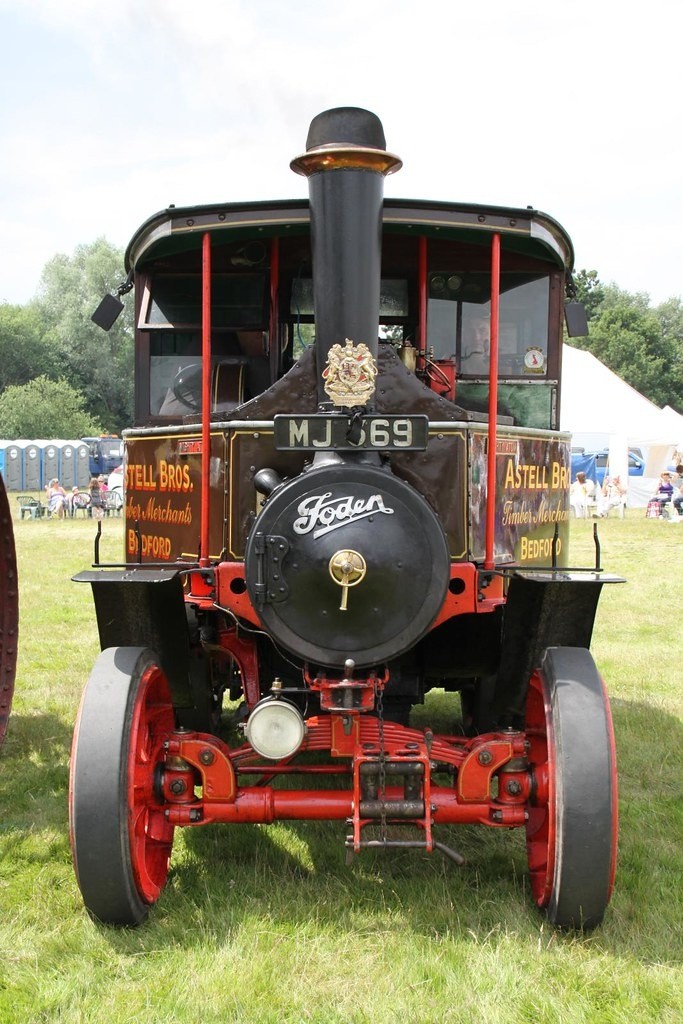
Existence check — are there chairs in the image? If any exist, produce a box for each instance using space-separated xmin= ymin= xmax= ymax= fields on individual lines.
xmin=574 ymin=491 xmax=629 ymax=520
xmin=16 ymin=485 xmax=124 ymax=520
xmin=648 ymin=487 xmax=683 ymax=519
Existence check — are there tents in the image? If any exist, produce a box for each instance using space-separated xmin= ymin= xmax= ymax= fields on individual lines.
xmin=562 ymin=341 xmax=683 ymax=457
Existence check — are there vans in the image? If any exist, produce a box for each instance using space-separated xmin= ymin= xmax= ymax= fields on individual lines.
xmin=81 ymin=435 xmax=125 ymax=474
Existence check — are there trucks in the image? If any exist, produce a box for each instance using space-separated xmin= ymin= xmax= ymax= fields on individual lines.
xmin=570 ymin=452 xmax=675 ymax=488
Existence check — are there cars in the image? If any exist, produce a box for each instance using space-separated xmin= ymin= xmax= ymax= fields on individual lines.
xmin=67 ymin=106 xmax=626 ymax=931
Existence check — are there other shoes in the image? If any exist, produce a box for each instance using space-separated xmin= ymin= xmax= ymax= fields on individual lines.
xmin=593 ymin=513 xmax=602 ymax=519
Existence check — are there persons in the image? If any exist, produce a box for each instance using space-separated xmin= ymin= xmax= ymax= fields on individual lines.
xmin=44 ymin=479 xmax=66 ymax=521
xmin=442 ymin=307 xmax=520 ymax=375
xmin=97 ymin=475 xmax=109 ymax=492
xmin=592 ymin=475 xmax=628 ymax=518
xmin=673 ymin=466 xmax=683 ymax=515
xmin=655 ymin=472 xmax=673 ymax=513
xmin=86 ymin=479 xmax=102 ymax=518
xmin=570 ymin=471 xmax=590 ymax=519
xmin=71 ymin=487 xmax=86 ymax=519
